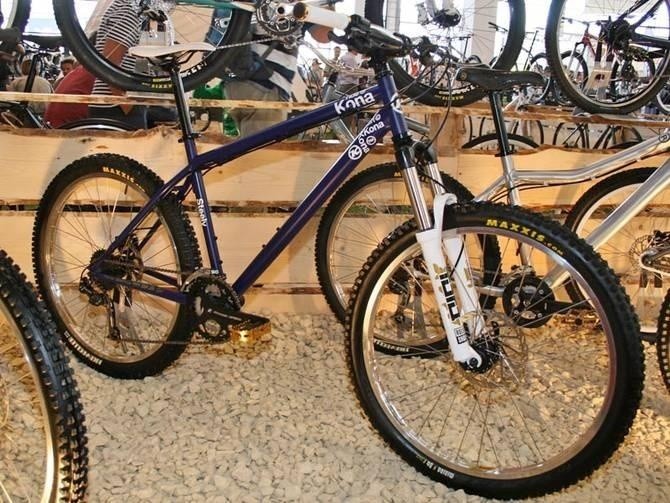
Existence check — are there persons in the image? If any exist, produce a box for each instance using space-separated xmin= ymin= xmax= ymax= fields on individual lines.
xmin=0 ymin=0 xmax=463 ymax=139
xmin=502 ymin=61 xmax=670 ymax=121
